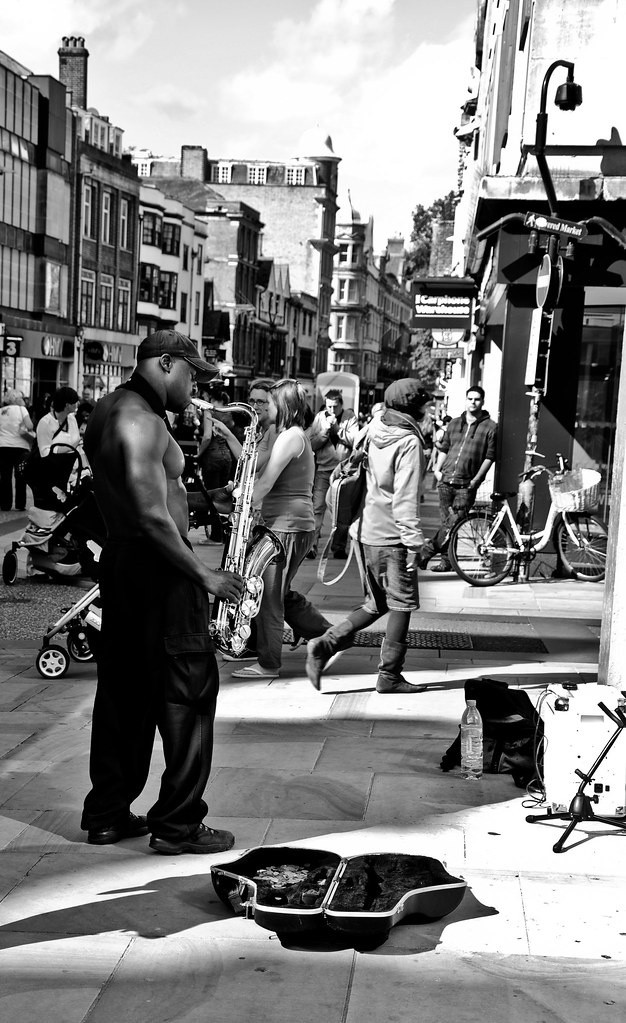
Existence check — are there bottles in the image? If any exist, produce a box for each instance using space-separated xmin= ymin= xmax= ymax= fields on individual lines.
xmin=459 ymin=700 xmax=484 ymax=781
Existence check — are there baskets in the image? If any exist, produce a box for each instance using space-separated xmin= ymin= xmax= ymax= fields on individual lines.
xmin=548 ymin=468 xmax=602 ymax=512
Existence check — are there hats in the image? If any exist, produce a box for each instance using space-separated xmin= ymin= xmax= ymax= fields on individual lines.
xmin=137 ymin=329 xmax=220 ymax=383
xmin=384 ymin=377 xmax=435 ymax=411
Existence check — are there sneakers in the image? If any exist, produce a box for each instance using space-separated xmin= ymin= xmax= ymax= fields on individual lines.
xmin=289 ymin=630 xmax=304 ymax=651
xmin=87 ymin=811 xmax=148 ymax=845
xmin=149 ymin=822 xmax=235 ymax=854
xmin=221 ymin=648 xmax=259 ymax=661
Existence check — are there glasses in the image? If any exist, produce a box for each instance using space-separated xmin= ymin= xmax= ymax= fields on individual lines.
xmin=248 ymin=398 xmax=268 ymax=406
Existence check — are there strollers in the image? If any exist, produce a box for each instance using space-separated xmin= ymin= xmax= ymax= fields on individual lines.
xmin=2 ymin=443 xmax=109 ymax=586
xmin=35 ymin=584 xmax=101 ymax=680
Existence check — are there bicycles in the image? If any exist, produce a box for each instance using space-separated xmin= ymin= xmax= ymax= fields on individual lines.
xmin=447 ymin=450 xmax=608 ymax=587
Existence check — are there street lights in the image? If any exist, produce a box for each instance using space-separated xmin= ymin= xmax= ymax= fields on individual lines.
xmin=512 ymin=59 xmax=583 ymax=588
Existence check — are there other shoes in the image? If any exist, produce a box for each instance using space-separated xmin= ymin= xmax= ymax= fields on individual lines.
xmin=419 ymin=552 xmax=428 ymax=570
xmin=306 ymin=549 xmax=316 ymax=559
xmin=430 ymin=562 xmax=454 ymax=572
xmin=334 ymin=551 xmax=347 ymax=559
xmin=198 ymin=539 xmax=223 ymax=545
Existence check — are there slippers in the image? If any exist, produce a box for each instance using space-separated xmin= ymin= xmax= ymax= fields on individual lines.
xmin=322 ymin=652 xmax=341 ymax=671
xmin=230 ymin=666 xmax=280 ymax=678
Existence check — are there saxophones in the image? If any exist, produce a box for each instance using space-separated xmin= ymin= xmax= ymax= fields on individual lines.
xmin=187 ymin=394 xmax=289 ymax=662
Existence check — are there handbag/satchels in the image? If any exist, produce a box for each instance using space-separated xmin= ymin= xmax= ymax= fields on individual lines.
xmin=439 ymin=678 xmax=544 ymax=774
xmin=325 ymin=427 xmax=370 ymax=531
xmin=19 ymin=422 xmax=36 ymax=443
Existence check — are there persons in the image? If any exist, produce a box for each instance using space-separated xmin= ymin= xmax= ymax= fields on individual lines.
xmin=306 ymin=378 xmax=434 ymax=694
xmin=81 ymin=331 xmax=244 ymax=856
xmin=207 ymin=379 xmax=335 ymax=677
xmin=310 ymin=390 xmax=453 ymax=559
xmin=189 ymin=386 xmax=235 ymax=546
xmin=417 ymin=386 xmax=498 ymax=572
xmin=0 ymin=389 xmax=33 ymax=512
xmin=36 ymin=387 xmax=92 ymax=490
xmin=74 ymin=388 xmax=97 ymax=437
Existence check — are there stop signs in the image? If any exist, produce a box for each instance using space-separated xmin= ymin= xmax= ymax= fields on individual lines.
xmin=536 ymin=254 xmax=551 ymax=308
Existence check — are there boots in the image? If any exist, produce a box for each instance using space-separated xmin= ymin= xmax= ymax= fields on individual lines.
xmin=304 ymin=618 xmax=355 ymax=691
xmin=375 ymin=637 xmax=427 ymax=693
